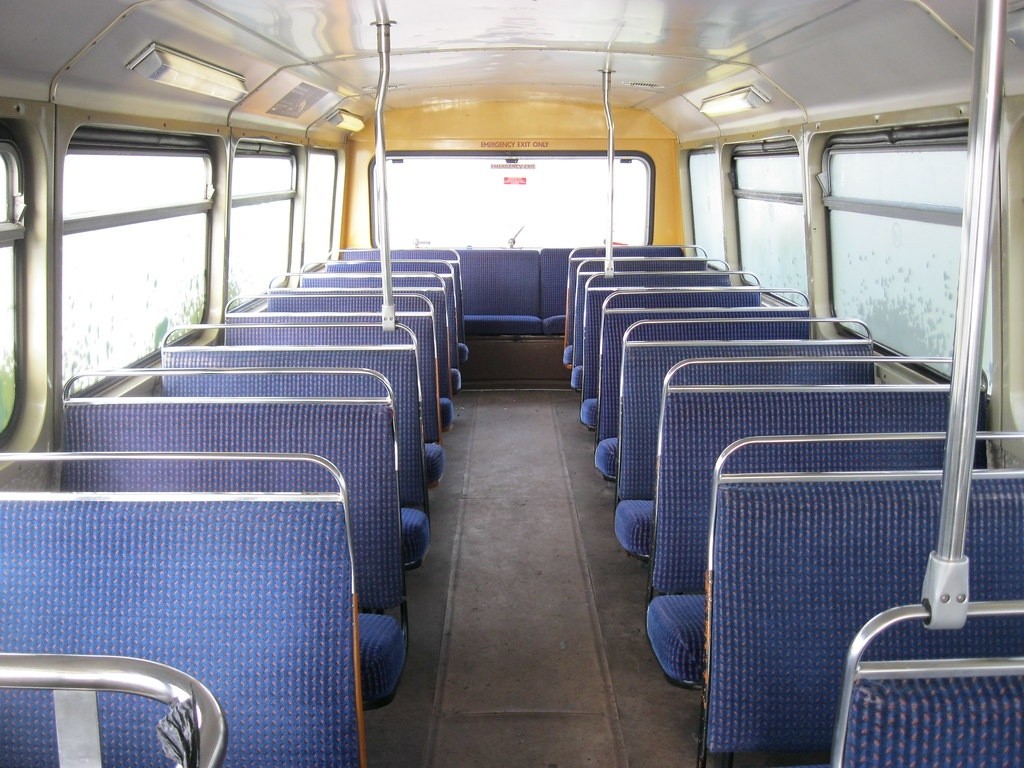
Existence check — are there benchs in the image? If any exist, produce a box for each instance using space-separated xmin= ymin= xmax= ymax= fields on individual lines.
xmin=324 ymin=248 xmax=469 ymax=365
xmin=612 ymin=317 xmax=875 ymax=561
xmin=300 ymin=259 xmax=462 ymax=396
xmin=268 ymin=270 xmax=454 ymax=435
xmin=56 ymin=368 xmax=412 ymax=710
xmin=570 ymin=257 xmax=732 ymax=393
xmin=456 ymin=249 xmax=542 ymax=335
xmin=222 ymin=290 xmax=445 ymax=486
xmin=645 ymin=357 xmax=989 ymax=690
xmin=0 ymin=451 xmax=369 ymax=768
xmin=592 ymin=288 xmax=812 ymax=481
xmin=159 ymin=322 xmax=434 ymax=568
xmin=540 ymin=247 xmax=687 ymax=335
xmin=579 ymin=271 xmax=761 ymax=431
xmin=562 ymin=244 xmax=709 ymax=369
xmin=698 ymin=430 xmax=1024 ymax=768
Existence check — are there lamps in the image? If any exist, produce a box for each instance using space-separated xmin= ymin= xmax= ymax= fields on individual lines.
xmin=123 ymin=42 xmax=248 ymax=104
xmin=325 ymin=109 xmax=365 ymax=134
xmin=698 ymin=86 xmax=770 ymax=118
